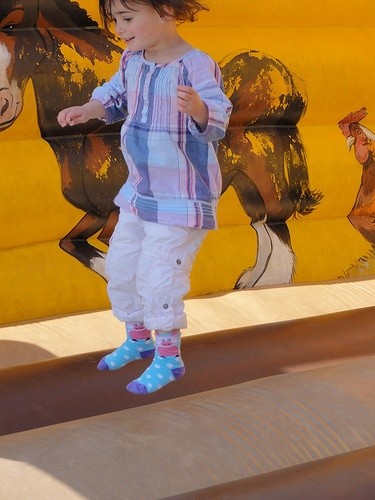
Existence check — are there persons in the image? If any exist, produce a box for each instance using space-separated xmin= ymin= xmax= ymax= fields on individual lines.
xmin=56 ymin=1 xmax=233 ymax=396
